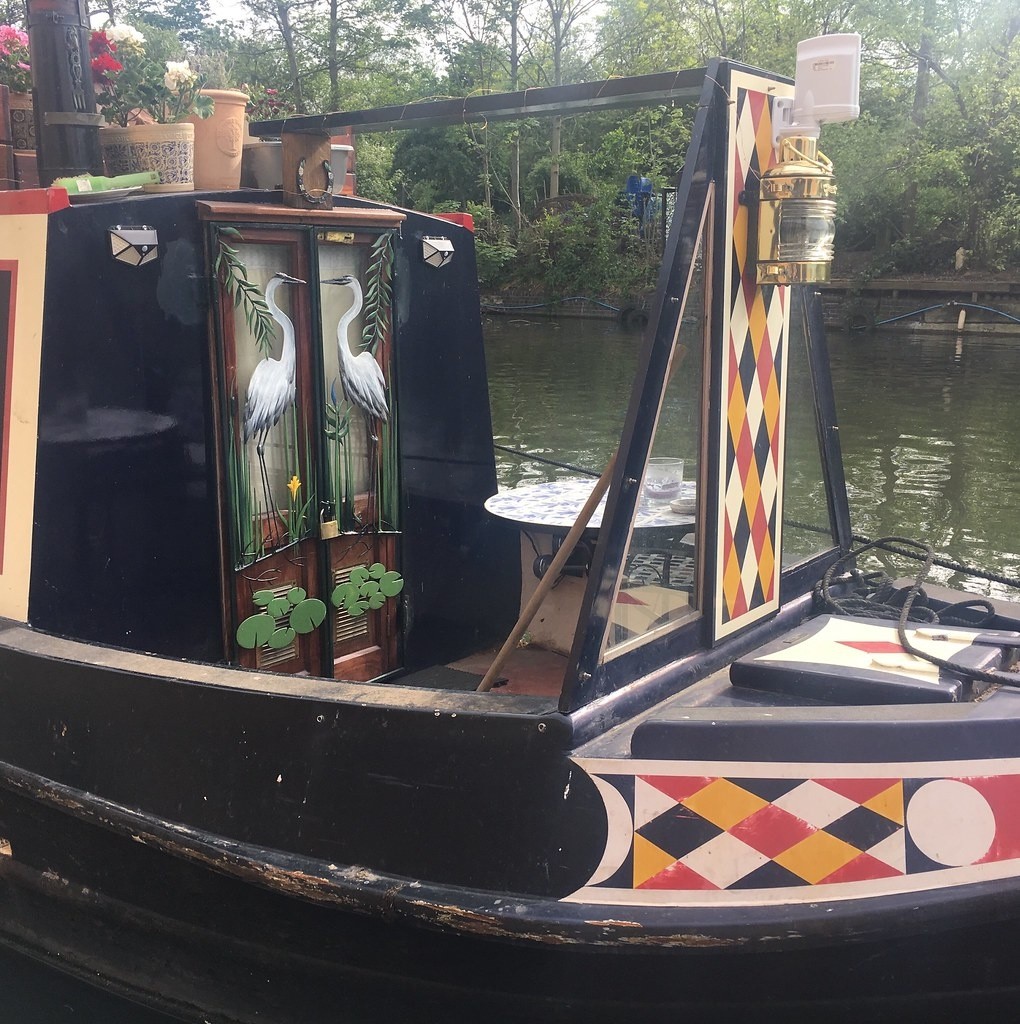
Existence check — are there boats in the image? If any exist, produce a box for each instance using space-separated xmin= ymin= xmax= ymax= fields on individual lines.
xmin=0 ymin=0 xmax=1020 ymax=1024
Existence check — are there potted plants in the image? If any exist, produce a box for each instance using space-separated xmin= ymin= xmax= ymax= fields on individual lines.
xmin=239 ymin=81 xmax=298 ymax=190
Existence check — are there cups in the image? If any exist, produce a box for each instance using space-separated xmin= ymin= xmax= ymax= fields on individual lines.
xmin=645 ymin=458 xmax=684 ymax=503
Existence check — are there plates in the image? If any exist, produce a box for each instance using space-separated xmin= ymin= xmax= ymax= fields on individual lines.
xmin=67 ymin=186 xmax=142 ymax=201
xmin=670 ymin=499 xmax=695 ymax=513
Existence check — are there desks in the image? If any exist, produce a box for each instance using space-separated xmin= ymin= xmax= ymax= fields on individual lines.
xmin=482 ymin=475 xmax=699 ymax=588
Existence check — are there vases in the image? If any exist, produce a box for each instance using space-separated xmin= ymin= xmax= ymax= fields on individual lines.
xmin=97 ymin=123 xmax=195 ymax=193
xmin=179 ymin=87 xmax=248 ymax=192
xmin=328 ymin=144 xmax=356 ymax=195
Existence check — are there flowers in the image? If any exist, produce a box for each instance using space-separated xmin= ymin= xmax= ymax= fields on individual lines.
xmin=0 ymin=24 xmax=37 ymax=97
xmin=87 ymin=23 xmax=214 ymax=129
xmin=176 ymin=47 xmax=243 ymax=93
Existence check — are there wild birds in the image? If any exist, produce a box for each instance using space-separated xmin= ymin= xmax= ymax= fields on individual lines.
xmin=241 ymin=270 xmax=308 ymax=569
xmin=319 ymin=273 xmax=398 ymax=564
